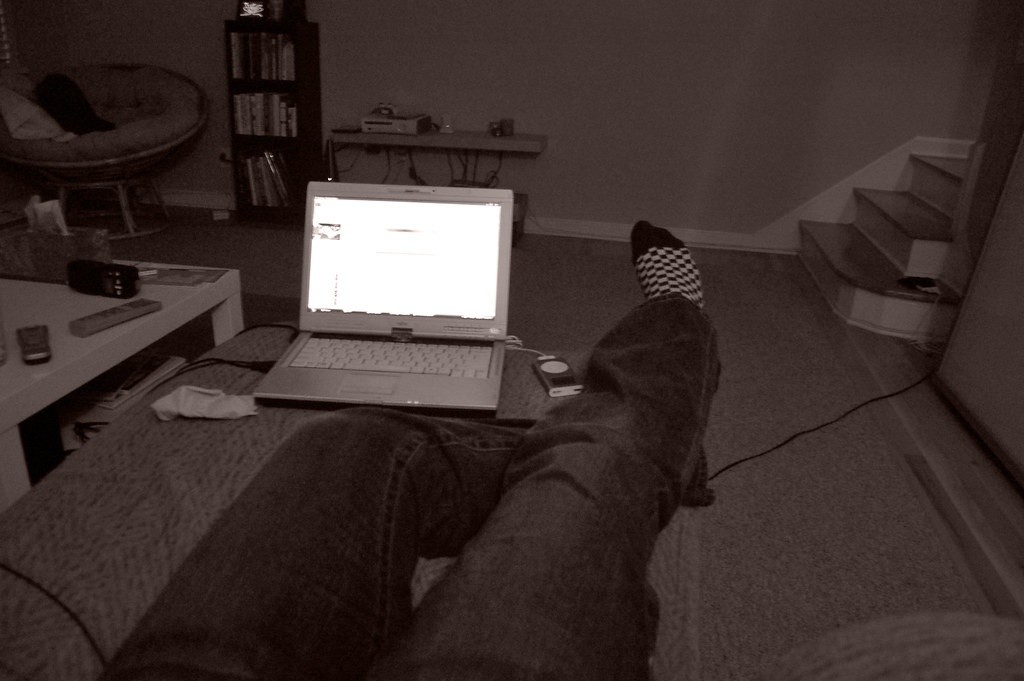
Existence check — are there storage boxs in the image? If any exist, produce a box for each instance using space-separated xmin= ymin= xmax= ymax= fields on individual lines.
xmin=512 ymin=193 xmax=528 ymax=221
xmin=512 ymin=219 xmax=525 ymax=247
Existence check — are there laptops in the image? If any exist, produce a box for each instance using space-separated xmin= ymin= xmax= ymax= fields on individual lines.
xmin=253 ymin=182 xmax=515 ymax=418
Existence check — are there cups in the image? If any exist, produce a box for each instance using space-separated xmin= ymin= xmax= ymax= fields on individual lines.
xmin=489 ymin=121 xmax=502 ymax=137
xmin=501 ymin=119 xmax=514 ymax=136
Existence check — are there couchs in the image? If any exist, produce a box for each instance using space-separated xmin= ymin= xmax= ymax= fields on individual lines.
xmin=0 ymin=321 xmax=703 ymax=681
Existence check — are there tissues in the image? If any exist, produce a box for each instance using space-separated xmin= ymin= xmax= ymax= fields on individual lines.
xmin=0 ymin=194 xmax=114 ymax=285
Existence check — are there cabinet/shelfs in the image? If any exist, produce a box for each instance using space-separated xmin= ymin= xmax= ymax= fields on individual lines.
xmin=225 ymin=19 xmax=323 ymax=227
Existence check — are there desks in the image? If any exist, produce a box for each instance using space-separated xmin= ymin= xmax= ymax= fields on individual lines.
xmin=333 ymin=126 xmax=549 ymax=188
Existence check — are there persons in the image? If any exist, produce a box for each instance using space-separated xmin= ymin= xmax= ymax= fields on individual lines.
xmin=96 ymin=220 xmax=722 ymax=681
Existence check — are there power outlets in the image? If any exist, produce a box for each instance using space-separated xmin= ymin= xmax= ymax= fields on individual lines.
xmin=221 ymin=146 xmax=231 ymax=168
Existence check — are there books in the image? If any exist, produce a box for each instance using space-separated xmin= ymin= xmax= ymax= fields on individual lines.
xmin=238 ymin=146 xmax=294 ymax=208
xmin=233 ymin=92 xmax=297 ymax=137
xmin=229 ymin=31 xmax=296 ymax=80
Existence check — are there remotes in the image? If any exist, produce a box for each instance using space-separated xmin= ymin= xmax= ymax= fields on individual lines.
xmin=68 ymin=298 xmax=161 ymax=338
xmin=15 ymin=324 xmax=52 ymax=366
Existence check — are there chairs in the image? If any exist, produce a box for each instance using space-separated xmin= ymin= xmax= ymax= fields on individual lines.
xmin=0 ymin=62 xmax=211 ymax=242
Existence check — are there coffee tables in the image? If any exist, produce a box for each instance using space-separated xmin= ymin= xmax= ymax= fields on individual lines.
xmin=0 ymin=259 xmax=246 ymax=514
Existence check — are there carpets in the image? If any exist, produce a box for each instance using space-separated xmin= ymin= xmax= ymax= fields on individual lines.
xmin=156 ymin=291 xmax=300 ymax=365
